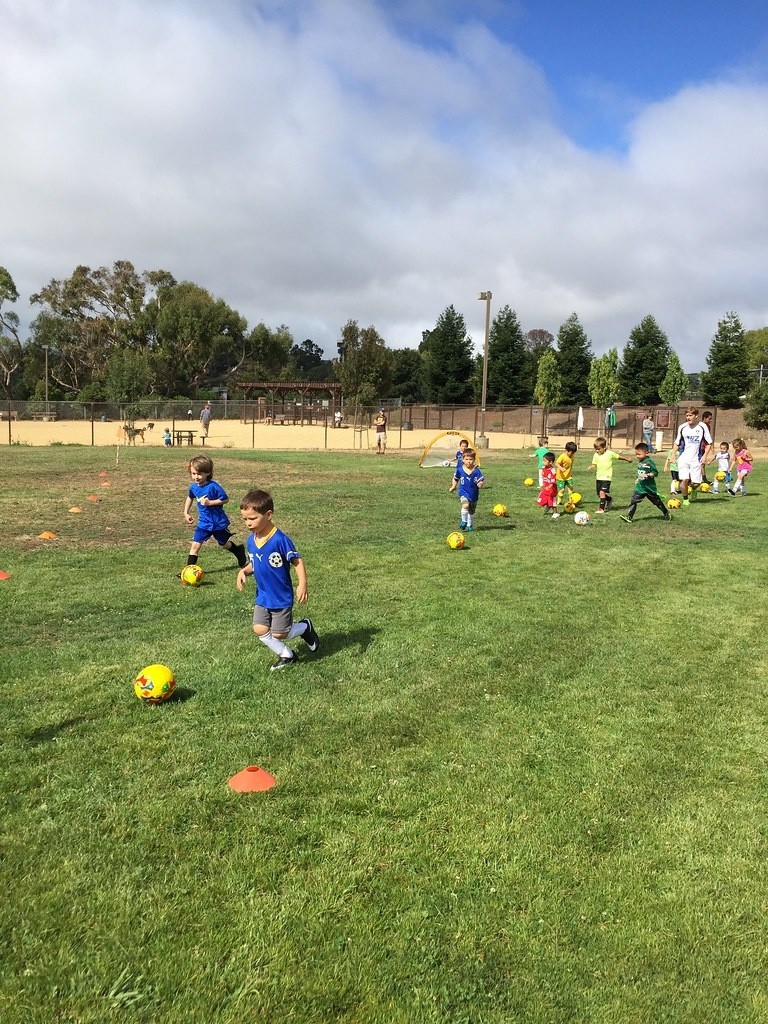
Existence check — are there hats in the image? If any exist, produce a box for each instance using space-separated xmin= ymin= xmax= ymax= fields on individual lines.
xmin=647 ymin=413 xmax=653 ymax=418
xmin=205 ymin=405 xmax=209 ymax=409
xmin=380 ymin=408 xmax=385 ymax=411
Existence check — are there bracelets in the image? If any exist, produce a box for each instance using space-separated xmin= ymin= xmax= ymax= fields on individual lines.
xmin=647 ymin=474 xmax=650 ymax=477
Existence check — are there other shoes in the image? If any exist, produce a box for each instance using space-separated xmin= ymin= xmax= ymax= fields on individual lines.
xmin=577 ymin=504 xmax=585 ymax=508
xmin=239 ymin=544 xmax=246 ymax=567
xmin=543 ymin=507 xmax=550 ymax=515
xmin=177 ymin=573 xmax=180 ymax=578
xmin=710 ymin=489 xmax=718 ymax=493
xmin=691 ymin=488 xmax=697 ymax=502
xmin=683 ymin=499 xmax=690 ymax=507
xmin=620 ymin=515 xmax=633 ymax=523
xmin=665 ymin=512 xmax=671 ymax=523
xmin=381 ymin=452 xmax=384 ymax=454
xmin=557 ymin=495 xmax=562 ymax=503
xmin=726 ymin=487 xmax=730 ymax=492
xmin=376 ymin=452 xmax=380 ymax=454
xmin=727 ymin=489 xmax=735 ymax=496
xmin=552 ymin=513 xmax=560 ymax=519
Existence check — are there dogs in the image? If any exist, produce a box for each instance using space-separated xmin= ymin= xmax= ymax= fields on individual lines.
xmin=123 ymin=426 xmax=145 ymax=444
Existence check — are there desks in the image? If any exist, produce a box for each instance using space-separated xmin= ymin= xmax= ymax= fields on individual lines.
xmin=171 ymin=429 xmax=198 ymax=446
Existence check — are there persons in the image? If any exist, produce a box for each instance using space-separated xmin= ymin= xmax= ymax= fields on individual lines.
xmin=331 ymin=409 xmax=341 ymax=428
xmin=176 ymin=455 xmax=248 ymax=577
xmin=620 ymin=443 xmax=671 ymax=523
xmin=188 ymin=407 xmax=194 ymax=421
xmin=664 ymin=406 xmax=753 ymax=506
xmin=529 ymin=438 xmax=551 ymax=488
xmin=555 ymin=442 xmax=583 ymax=508
xmin=642 ymin=414 xmax=657 ymax=454
xmin=200 ymin=405 xmax=212 ymax=438
xmin=236 ymin=490 xmax=320 ymax=671
xmin=536 ymin=452 xmax=561 ymax=519
xmin=586 ymin=437 xmax=633 ymax=514
xmin=162 ymin=428 xmax=172 ymax=447
xmin=264 ymin=410 xmax=273 ymax=425
xmin=373 ymin=408 xmax=387 ymax=454
xmin=447 ymin=439 xmax=484 ymax=532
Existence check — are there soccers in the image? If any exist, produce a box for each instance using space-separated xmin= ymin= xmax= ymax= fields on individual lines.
xmin=715 ymin=471 xmax=726 ymax=481
xmin=134 ymin=664 xmax=177 ymax=704
xmin=181 ymin=564 xmax=204 ymax=586
xmin=675 ymin=481 xmax=710 ymax=495
xmin=442 ymin=460 xmax=450 ymax=467
xmin=564 ymin=502 xmax=576 ymax=514
xmin=446 ymin=531 xmax=465 ymax=550
xmin=569 ymin=493 xmax=582 ymax=506
xmin=493 ymin=504 xmax=507 ymax=516
xmin=524 ymin=478 xmax=534 ymax=487
xmin=574 ymin=511 xmax=590 ymax=526
xmin=667 ymin=498 xmax=681 ymax=509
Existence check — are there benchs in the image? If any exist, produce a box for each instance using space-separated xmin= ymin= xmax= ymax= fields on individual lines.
xmin=285 ymin=415 xmax=318 ymax=426
xmin=174 ymin=435 xmax=207 ymax=446
xmin=324 ymin=415 xmax=349 ymax=428
xmin=269 ymin=414 xmax=286 ymax=424
xmin=30 ymin=412 xmax=58 ymax=421
xmin=0 ymin=411 xmax=18 ymax=421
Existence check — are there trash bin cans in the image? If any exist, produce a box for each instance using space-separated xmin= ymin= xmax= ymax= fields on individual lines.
xmin=403 ymin=422 xmax=413 ymax=429
xmin=101 ymin=416 xmax=106 ymax=422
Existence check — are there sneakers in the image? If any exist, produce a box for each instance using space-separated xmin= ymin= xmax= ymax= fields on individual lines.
xmin=299 ymin=618 xmax=319 ymax=652
xmin=459 ymin=521 xmax=467 ymax=530
xmin=465 ymin=526 xmax=472 ymax=532
xmin=270 ymin=649 xmax=300 ymax=670
xmin=594 ymin=508 xmax=605 ymax=514
xmin=605 ymin=496 xmax=613 ymax=512
